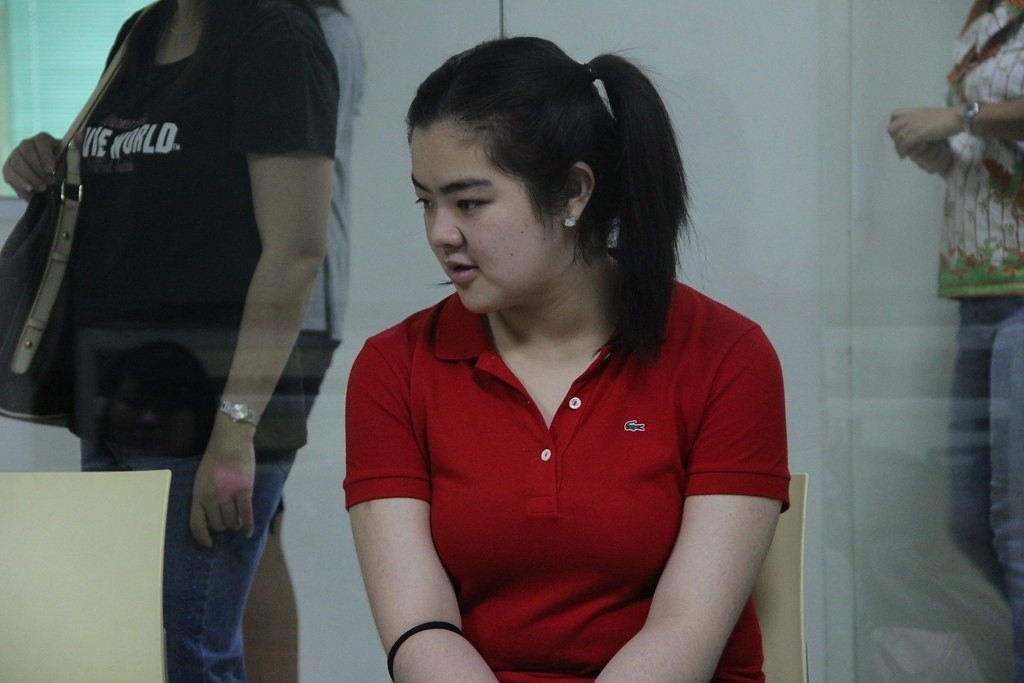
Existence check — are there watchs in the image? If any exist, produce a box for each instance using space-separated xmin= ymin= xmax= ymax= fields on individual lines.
xmin=219 ymin=397 xmax=259 ymax=431
xmin=961 ymin=98 xmax=983 ymax=134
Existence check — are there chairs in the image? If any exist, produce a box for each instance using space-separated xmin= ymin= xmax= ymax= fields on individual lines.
xmin=751 ymin=472 xmax=809 ymax=683
xmin=0 ymin=466 xmax=174 ymax=683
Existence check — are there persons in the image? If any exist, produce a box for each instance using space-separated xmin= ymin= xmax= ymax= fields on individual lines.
xmin=886 ymin=1 xmax=1024 ymax=682
xmin=2 ymin=0 xmax=364 ymax=682
xmin=340 ymin=33 xmax=791 ymax=682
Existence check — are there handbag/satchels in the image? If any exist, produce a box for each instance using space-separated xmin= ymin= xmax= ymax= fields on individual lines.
xmin=0 ymin=0 xmax=161 ymax=422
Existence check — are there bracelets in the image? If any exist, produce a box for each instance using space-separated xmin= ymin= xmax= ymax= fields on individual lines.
xmin=386 ymin=620 xmax=466 ymax=681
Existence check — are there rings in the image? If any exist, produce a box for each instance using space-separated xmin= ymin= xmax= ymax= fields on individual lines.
xmin=897 ymin=128 xmax=907 ymax=142
